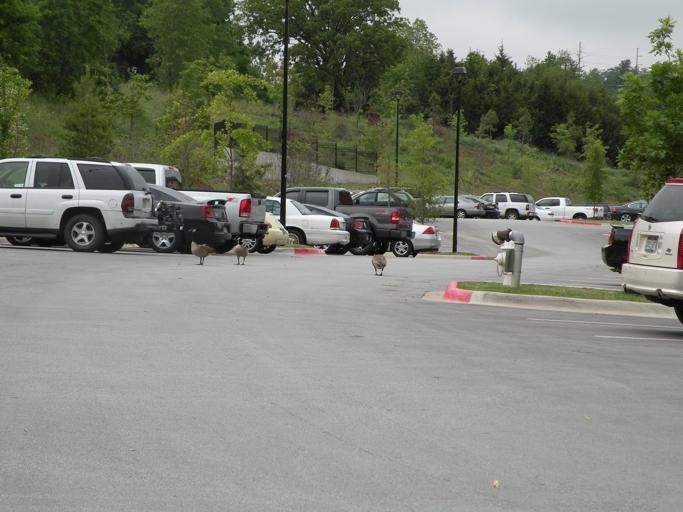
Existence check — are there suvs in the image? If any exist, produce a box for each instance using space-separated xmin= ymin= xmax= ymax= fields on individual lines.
xmin=350 ymin=187 xmax=417 ymax=219
xmin=478 ymin=192 xmax=535 ymax=220
xmin=1 ymin=155 xmax=184 ymax=254
xmin=620 ymin=177 xmax=683 ymax=325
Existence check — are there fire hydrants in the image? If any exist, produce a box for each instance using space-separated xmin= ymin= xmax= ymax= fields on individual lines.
xmin=494 ymin=240 xmax=517 ymax=287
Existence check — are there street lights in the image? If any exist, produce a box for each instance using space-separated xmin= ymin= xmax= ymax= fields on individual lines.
xmin=450 ymin=65 xmax=467 ymax=252
xmin=394 ymin=88 xmax=404 ymax=186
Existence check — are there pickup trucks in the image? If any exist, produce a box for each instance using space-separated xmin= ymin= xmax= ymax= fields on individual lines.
xmin=529 ymin=197 xmax=608 ymax=221
xmin=111 ymin=161 xmax=266 ymax=256
xmin=601 ymin=224 xmax=633 ymax=273
xmin=271 ymin=185 xmax=413 ymax=256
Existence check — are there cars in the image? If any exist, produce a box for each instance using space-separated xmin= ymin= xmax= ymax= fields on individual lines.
xmin=461 ymin=193 xmax=499 ymax=219
xmin=235 ymin=210 xmax=289 ymax=255
xmin=608 ymin=199 xmax=650 ymax=223
xmin=425 ymin=195 xmax=486 ymax=218
xmin=148 ymin=182 xmax=232 ymax=255
xmin=302 ymin=202 xmax=372 ymax=256
xmin=258 ymin=193 xmax=351 ymax=249
xmin=388 ymin=219 xmax=441 ymax=257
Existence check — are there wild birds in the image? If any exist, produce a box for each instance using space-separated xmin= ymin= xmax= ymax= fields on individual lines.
xmin=371 ymin=242 xmax=386 ymax=277
xmin=189 ymin=238 xmax=216 ymax=265
xmin=225 ymin=238 xmax=249 ymax=266
